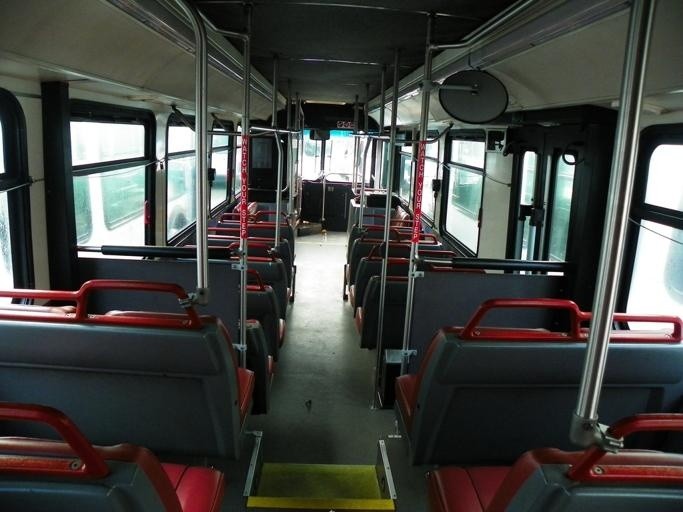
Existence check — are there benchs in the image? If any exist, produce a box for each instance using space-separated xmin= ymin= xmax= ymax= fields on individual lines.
xmin=0 ymin=202 xmax=297 ymax=511
xmin=343 ymin=204 xmax=683 ymax=511
